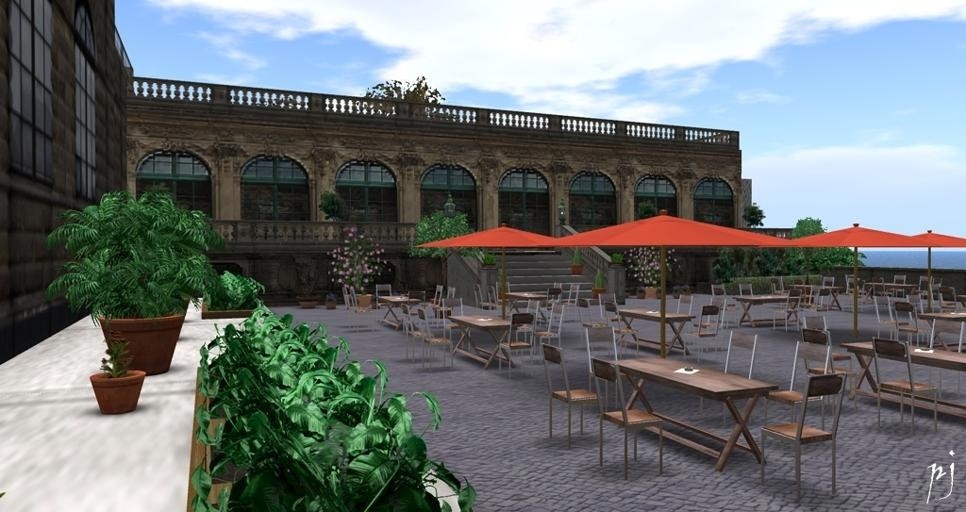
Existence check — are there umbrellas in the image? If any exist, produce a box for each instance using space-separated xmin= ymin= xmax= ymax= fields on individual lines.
xmin=786 ymin=221 xmax=907 ymax=338
xmin=556 ymin=208 xmax=798 ymax=361
xmin=898 ymin=229 xmax=966 ymax=314
xmin=414 ymin=223 xmax=556 ymax=320
